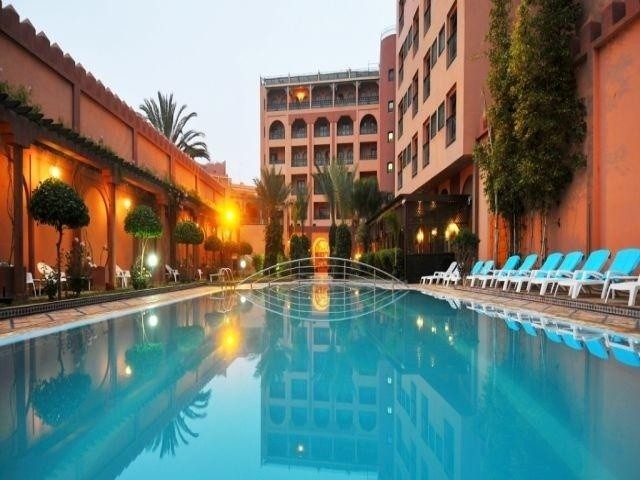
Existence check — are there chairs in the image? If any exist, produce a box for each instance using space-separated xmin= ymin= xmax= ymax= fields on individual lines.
xmin=115 ymin=264 xmax=132 ymax=289
xmin=165 ymin=264 xmax=181 ymax=282
xmin=25 ymin=261 xmax=67 ymax=297
xmin=418 ymin=246 xmax=639 ymax=308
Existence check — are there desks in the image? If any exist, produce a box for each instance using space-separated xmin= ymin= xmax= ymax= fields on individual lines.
xmin=209 ymin=273 xmax=220 ymax=283
